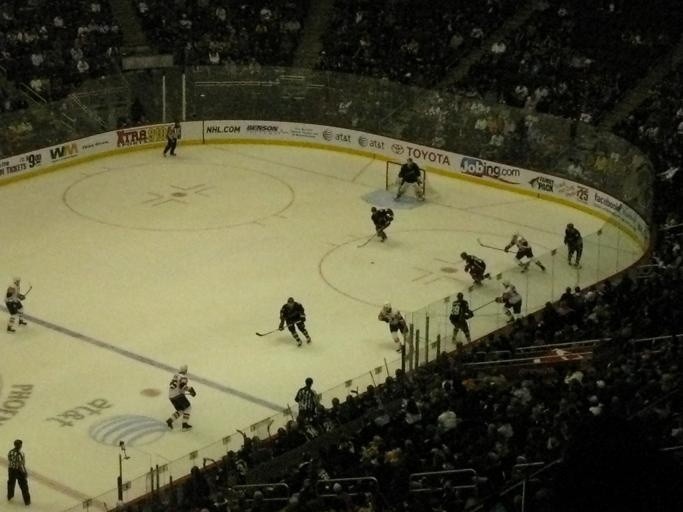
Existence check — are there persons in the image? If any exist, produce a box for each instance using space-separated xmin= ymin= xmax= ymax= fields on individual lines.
xmin=0 ymin=1 xmax=682 ymax=511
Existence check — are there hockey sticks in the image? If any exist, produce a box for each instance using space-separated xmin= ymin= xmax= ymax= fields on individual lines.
xmin=357 ymin=222 xmax=388 ymax=248
xmin=476 ymin=238 xmax=514 ymax=254
xmin=256 ymin=321 xmax=301 ymax=336
xmin=18 ymin=281 xmax=33 ymax=301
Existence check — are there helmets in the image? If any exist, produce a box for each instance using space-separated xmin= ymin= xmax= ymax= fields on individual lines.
xmin=380 ymin=221 xmax=574 ymax=314
xmin=13 ymin=276 xmax=20 ymax=283
xmin=287 ymin=296 xmax=294 ymax=303
xmin=174 ymin=120 xmax=180 ymax=125
xmin=179 ymin=364 xmax=189 ymax=373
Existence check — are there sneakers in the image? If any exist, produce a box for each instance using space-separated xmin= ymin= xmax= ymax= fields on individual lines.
xmin=7 ymin=321 xmax=27 ymax=332
xmin=166 ymin=418 xmax=193 ymax=429
xmin=376 ymin=234 xmax=388 ymax=242
xmin=297 ymin=337 xmax=312 ymax=347
xmin=389 ymin=317 xmax=516 ymax=352
xmin=161 ymin=151 xmax=178 ymax=159
xmin=393 ymin=194 xmax=428 ymax=202
